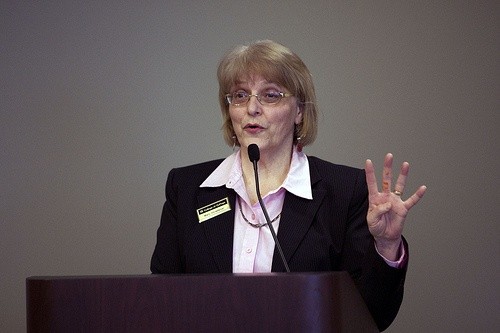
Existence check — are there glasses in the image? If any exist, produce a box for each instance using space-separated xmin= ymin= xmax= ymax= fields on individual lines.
xmin=224 ymin=89 xmax=293 ymax=106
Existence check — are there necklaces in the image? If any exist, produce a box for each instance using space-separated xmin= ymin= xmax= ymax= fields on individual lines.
xmin=236 ymin=194 xmax=282 ymax=228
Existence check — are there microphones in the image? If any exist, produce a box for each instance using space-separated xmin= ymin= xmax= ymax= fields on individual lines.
xmin=247 ymin=144 xmax=290 ymax=273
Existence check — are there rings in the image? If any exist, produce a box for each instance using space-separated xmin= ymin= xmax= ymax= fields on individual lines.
xmin=393 ymin=190 xmax=403 ymax=196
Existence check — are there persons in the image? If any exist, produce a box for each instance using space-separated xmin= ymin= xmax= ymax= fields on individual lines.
xmin=149 ymin=38 xmax=428 ymax=333
